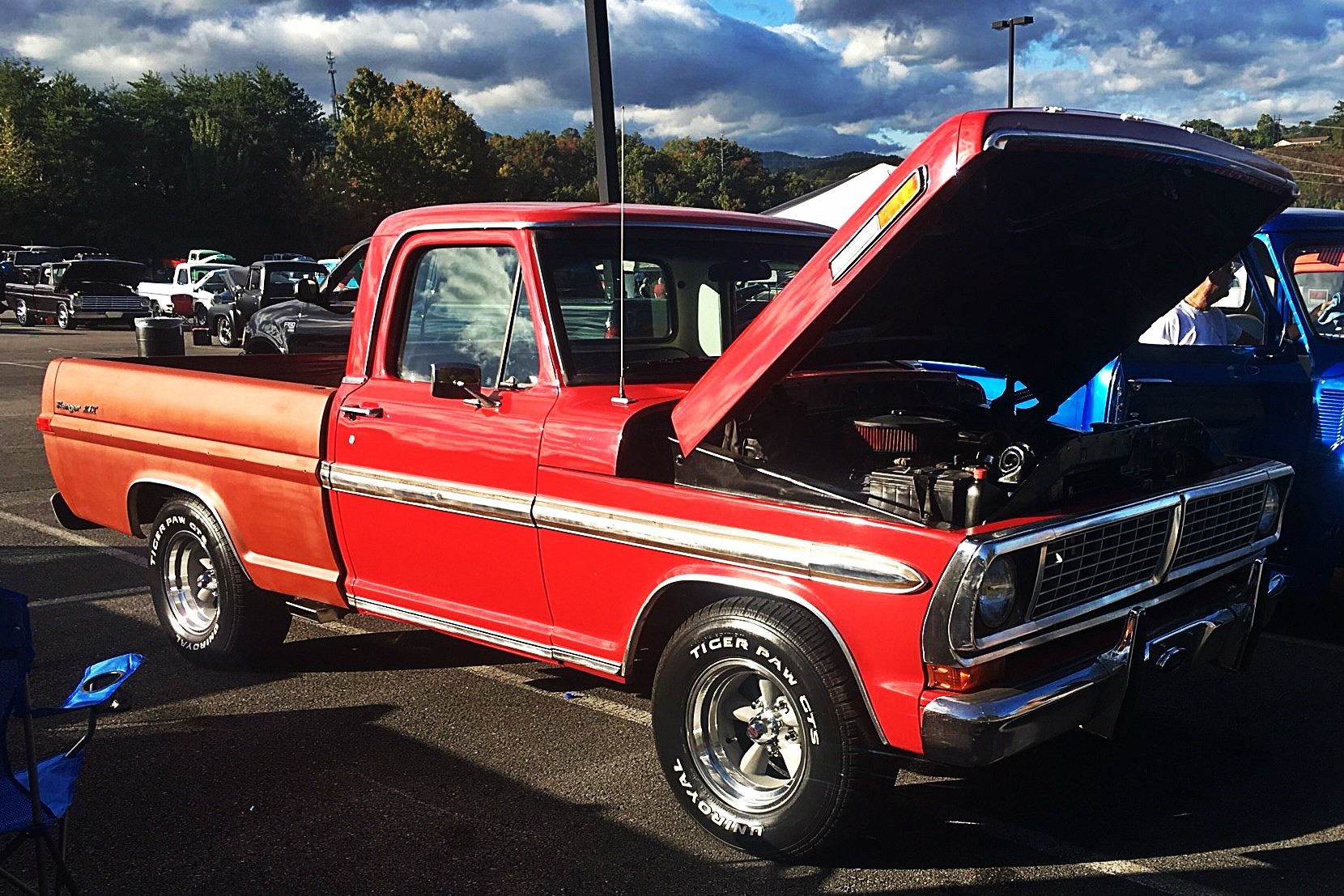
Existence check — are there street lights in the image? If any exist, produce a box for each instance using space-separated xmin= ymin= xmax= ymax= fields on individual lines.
xmin=990 ymin=14 xmax=1034 ymax=108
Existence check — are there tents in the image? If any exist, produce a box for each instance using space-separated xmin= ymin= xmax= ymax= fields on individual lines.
xmin=698 ymin=161 xmax=898 ymax=357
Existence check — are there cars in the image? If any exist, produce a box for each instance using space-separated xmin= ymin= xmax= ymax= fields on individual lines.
xmin=916 ymin=202 xmax=1344 ymax=643
xmin=0 ymin=238 xmax=673 ymax=352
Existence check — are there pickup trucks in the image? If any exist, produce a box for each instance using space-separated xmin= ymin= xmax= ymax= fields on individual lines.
xmin=35 ymin=107 xmax=1310 ymax=862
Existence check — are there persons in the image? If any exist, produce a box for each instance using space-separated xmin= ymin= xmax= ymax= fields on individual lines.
xmin=332 ymin=245 xmax=366 ymax=292
xmin=639 ymin=276 xmax=666 ymax=298
xmin=1139 ymin=261 xmax=1335 ymax=345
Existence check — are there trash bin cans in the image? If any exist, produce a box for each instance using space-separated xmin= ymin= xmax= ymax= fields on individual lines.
xmin=134 ymin=317 xmax=186 ymax=357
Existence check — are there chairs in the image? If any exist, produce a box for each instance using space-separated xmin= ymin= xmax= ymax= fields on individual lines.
xmin=0 ymin=588 xmax=146 ymax=896
